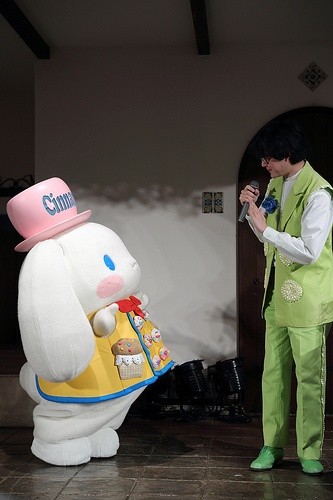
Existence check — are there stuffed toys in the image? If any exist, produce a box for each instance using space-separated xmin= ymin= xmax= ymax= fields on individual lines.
xmin=6 ymin=176 xmax=177 ymax=467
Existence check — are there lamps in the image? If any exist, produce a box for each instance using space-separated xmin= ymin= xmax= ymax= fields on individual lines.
xmin=172 ymin=357 xmax=249 ymax=419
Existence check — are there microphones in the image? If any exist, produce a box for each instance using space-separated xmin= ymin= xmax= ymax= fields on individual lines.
xmin=239 ymin=180 xmax=259 ymax=222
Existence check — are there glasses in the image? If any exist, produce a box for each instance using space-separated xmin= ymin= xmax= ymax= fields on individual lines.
xmin=259 ymin=156 xmax=273 ymax=164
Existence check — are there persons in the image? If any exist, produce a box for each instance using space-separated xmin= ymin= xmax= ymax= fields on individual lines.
xmin=238 ymin=132 xmax=333 ymax=476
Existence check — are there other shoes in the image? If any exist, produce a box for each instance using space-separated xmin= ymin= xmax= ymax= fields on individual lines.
xmin=298 ymin=456 xmax=324 ymax=474
xmin=250 ymin=446 xmax=285 ymax=471
